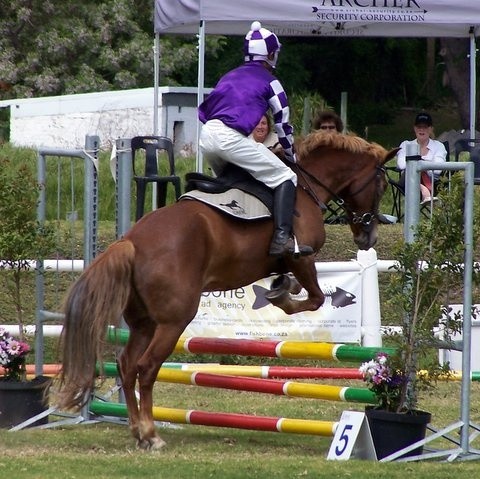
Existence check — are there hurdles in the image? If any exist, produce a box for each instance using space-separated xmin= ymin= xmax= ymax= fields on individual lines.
xmin=83 ymin=135 xmax=473 ymax=436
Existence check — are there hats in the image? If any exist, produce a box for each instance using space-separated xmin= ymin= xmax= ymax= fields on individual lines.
xmin=244 ymin=21 xmax=281 ymax=56
xmin=414 ymin=114 xmax=432 ymax=126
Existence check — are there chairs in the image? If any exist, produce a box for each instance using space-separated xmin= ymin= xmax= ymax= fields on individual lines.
xmin=326 ymin=204 xmax=345 ymax=225
xmin=382 ymin=141 xmax=450 ymax=223
xmin=131 ymin=134 xmax=183 ymax=223
xmin=442 ymin=138 xmax=480 ymax=222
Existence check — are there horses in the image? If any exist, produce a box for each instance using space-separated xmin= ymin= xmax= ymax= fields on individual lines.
xmin=54 ymin=128 xmax=401 ymax=451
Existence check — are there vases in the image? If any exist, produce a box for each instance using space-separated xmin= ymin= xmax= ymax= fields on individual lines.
xmin=363 ymin=407 xmax=432 ymax=463
xmin=0 ymin=376 xmax=52 ymax=431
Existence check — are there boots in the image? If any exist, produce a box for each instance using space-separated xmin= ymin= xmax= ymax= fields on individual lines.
xmin=268 ymin=180 xmax=313 ymax=257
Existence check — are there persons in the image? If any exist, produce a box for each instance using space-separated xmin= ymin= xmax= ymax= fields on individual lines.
xmin=248 ymin=112 xmax=282 ymax=150
xmin=397 ymin=114 xmax=447 ymax=205
xmin=311 ymin=110 xmax=343 ymax=133
xmin=197 ymin=20 xmax=313 ymax=256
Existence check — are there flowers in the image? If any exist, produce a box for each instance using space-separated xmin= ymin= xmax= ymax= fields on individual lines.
xmin=0 ymin=330 xmax=36 ymax=381
xmin=358 ymin=353 xmax=434 ymax=412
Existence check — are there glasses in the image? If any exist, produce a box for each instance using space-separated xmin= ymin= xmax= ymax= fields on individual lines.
xmin=320 ymin=126 xmax=334 ymax=130
